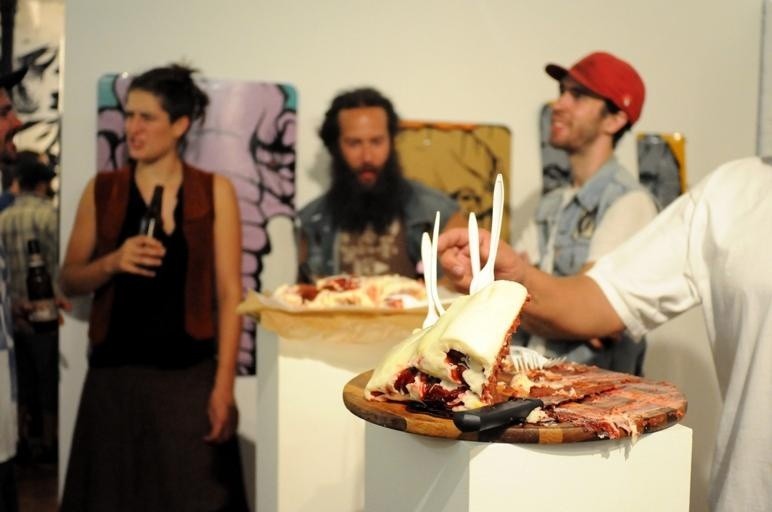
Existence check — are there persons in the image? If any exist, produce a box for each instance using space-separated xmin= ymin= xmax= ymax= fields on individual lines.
xmin=5 ymin=151 xmax=40 ymax=193
xmin=293 ymin=88 xmax=464 ymax=283
xmin=512 ymin=54 xmax=657 ymax=377
xmin=0 ymin=162 xmax=58 ymax=476
xmin=437 ymin=156 xmax=772 ymax=512
xmin=58 ymin=62 xmax=255 ymax=511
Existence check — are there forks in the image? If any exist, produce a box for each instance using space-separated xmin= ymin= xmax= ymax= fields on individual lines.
xmin=503 ymin=344 xmax=564 ymax=372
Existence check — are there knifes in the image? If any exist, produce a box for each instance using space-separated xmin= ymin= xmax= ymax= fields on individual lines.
xmin=451 ymin=362 xmax=621 ymax=433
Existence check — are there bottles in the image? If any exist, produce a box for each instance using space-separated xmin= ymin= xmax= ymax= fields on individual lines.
xmin=24 ymin=237 xmax=60 ymax=337
xmin=135 ymin=185 xmax=163 ymax=246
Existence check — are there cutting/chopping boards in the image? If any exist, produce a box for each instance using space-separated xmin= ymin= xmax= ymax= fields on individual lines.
xmin=343 ymin=360 xmax=687 ymax=444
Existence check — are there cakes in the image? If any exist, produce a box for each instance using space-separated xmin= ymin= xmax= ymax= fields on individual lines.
xmin=365 ymin=175 xmax=533 ymax=410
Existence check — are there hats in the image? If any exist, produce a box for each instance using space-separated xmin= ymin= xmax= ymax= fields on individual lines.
xmin=545 ymin=54 xmax=646 ymax=123
